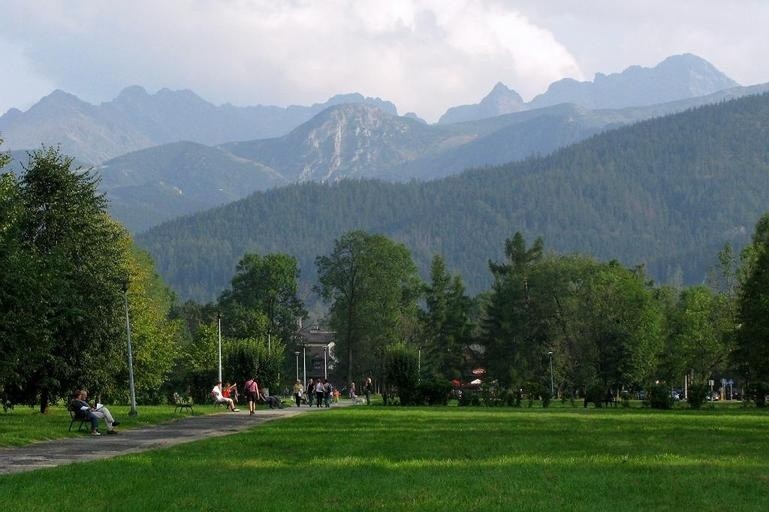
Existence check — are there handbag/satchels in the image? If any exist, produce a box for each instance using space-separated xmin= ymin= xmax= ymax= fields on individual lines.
xmin=327 ymin=385 xmax=332 ymax=392
xmin=244 ymin=388 xmax=250 ymax=396
xmin=297 ymin=392 xmax=301 ymax=397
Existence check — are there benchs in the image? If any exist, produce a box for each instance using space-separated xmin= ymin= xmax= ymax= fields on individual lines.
xmin=174 ymin=390 xmax=194 ymax=414
xmin=65 ymin=403 xmax=95 ymax=434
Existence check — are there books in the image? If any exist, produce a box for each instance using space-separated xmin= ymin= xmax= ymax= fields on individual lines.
xmin=94 ymin=403 xmax=103 ymax=411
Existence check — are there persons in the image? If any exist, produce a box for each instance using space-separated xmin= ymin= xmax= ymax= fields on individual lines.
xmin=222 ymin=382 xmax=236 ymax=409
xmin=244 ymin=376 xmax=260 ymax=416
xmin=258 ymin=376 xmax=374 ymax=409
xmin=73 ymin=391 xmax=103 ymax=436
xmin=80 ymin=390 xmax=121 ymax=434
xmin=213 ymin=381 xmax=240 ymax=412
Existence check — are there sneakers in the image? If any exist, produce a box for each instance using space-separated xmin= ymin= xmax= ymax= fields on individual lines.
xmin=91 ymin=432 xmax=100 ymax=436
xmin=296 ymin=404 xmax=330 ymax=408
xmin=230 ymin=408 xmax=255 ymax=416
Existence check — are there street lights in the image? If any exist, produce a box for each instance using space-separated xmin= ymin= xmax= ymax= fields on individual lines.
xmin=322 ymin=346 xmax=327 ymax=381
xmin=302 ymin=341 xmax=308 ymax=390
xmin=547 ymin=351 xmax=555 ymax=397
xmin=294 ymin=350 xmax=300 ymax=381
xmin=418 ymin=346 xmax=423 ymax=382
xmin=266 ymin=326 xmax=273 ymax=356
xmin=216 ymin=310 xmax=224 ymax=407
xmin=113 ymin=277 xmax=139 ymax=416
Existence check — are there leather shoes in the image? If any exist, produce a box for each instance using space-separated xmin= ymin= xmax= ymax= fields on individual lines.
xmin=107 ymin=431 xmax=118 ymax=434
xmin=111 ymin=422 xmax=119 ymax=426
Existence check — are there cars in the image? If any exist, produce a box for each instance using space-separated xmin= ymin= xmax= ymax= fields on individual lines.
xmin=619 ymin=388 xmax=741 ymax=401
